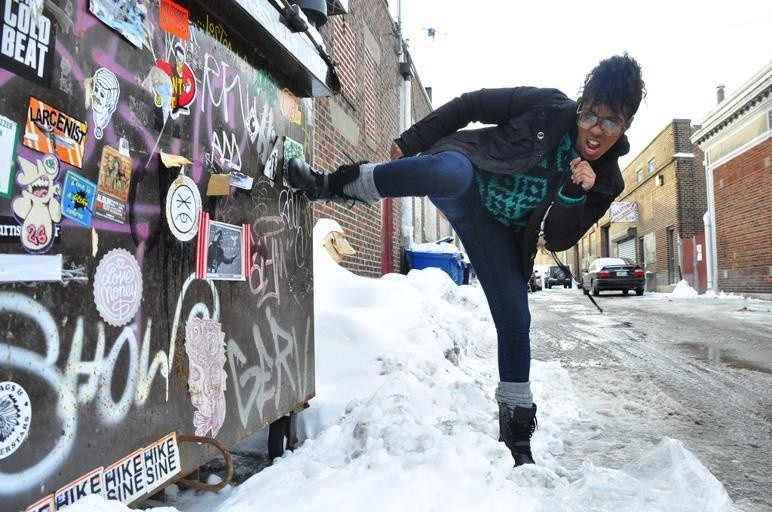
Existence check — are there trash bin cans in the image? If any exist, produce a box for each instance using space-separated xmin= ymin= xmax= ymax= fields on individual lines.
xmin=406 ymin=235 xmax=472 ymax=287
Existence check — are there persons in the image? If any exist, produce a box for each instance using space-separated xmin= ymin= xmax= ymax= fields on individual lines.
xmin=282 ymin=51 xmax=647 ymax=470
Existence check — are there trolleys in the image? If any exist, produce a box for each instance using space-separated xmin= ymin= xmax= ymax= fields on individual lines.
xmin=0 ymin=0 xmax=320 ymax=512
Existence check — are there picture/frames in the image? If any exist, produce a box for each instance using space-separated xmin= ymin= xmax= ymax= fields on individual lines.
xmin=205 ymin=218 xmax=247 ymax=283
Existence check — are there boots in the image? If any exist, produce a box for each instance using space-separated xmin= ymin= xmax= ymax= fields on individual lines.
xmin=498 ymin=400 xmax=541 ymax=468
xmin=288 ymin=158 xmax=370 ymax=212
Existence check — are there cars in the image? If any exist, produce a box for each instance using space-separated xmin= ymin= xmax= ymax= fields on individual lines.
xmin=528 ymin=269 xmax=543 ymax=291
xmin=580 ymin=256 xmax=646 ymax=298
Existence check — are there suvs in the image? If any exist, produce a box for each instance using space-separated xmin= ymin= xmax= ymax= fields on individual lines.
xmin=545 ymin=264 xmax=572 ymax=290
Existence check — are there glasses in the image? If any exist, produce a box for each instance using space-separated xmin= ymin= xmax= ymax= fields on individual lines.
xmin=576 ymin=99 xmax=627 ymax=138
